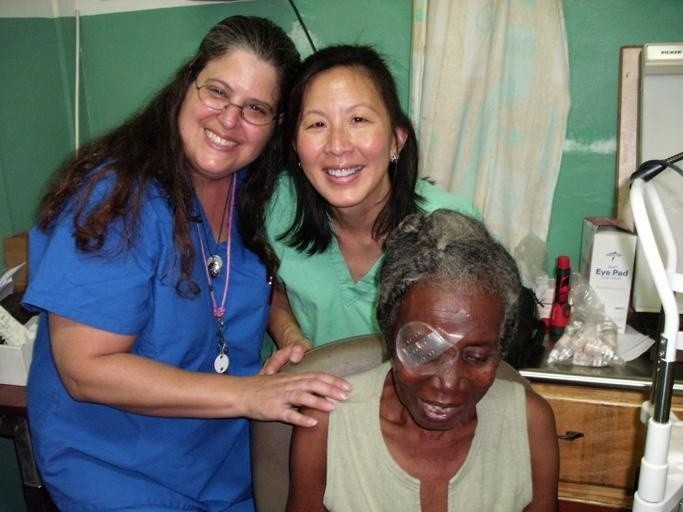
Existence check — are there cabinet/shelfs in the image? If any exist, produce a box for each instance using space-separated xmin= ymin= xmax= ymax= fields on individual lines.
xmin=529 ymin=383 xmax=682 ymax=509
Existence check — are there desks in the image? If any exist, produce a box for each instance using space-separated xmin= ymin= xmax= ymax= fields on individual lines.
xmin=0 ymin=383 xmax=53 ymax=512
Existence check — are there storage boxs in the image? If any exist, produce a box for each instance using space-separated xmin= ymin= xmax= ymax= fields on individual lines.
xmin=1 ymin=261 xmax=40 ymax=389
xmin=580 ymin=217 xmax=637 ymax=334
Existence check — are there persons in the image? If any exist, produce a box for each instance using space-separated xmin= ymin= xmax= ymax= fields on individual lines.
xmin=278 ymin=207 xmax=564 ymax=511
xmin=253 ymin=40 xmax=487 ymax=381
xmin=18 ymin=15 xmax=355 ymax=512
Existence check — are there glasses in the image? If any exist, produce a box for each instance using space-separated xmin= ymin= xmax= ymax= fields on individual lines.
xmin=191 ymin=75 xmax=283 ymax=127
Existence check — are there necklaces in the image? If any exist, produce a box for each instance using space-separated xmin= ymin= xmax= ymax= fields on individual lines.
xmin=175 ymin=173 xmax=240 ymax=375
xmin=182 ymin=175 xmax=236 ymax=280
